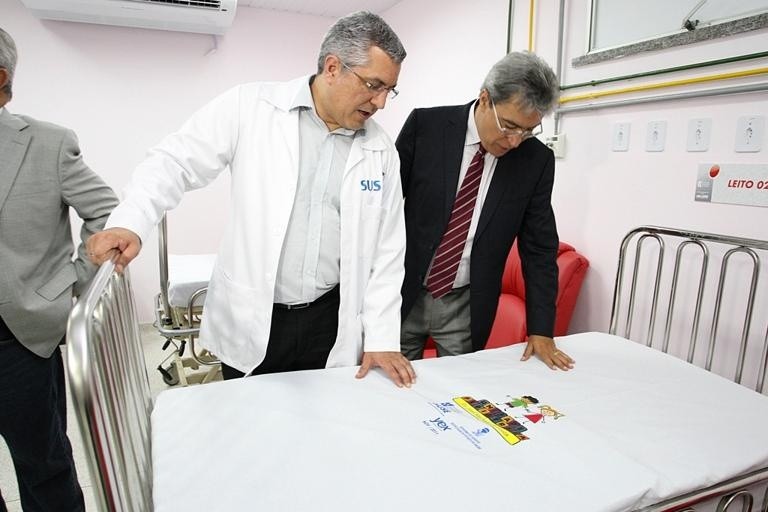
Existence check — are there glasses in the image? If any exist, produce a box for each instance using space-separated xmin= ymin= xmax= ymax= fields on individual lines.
xmin=491 ymin=98 xmax=542 ymax=137
xmin=335 ymin=55 xmax=399 ymax=99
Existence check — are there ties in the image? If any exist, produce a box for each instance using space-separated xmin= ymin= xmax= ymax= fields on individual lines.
xmin=426 ymin=142 xmax=487 ymax=298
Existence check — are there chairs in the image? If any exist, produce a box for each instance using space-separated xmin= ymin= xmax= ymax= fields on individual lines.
xmin=421 ymin=231 xmax=589 ymax=358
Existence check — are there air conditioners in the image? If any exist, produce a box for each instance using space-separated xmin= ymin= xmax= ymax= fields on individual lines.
xmin=20 ymin=0 xmax=239 ymax=37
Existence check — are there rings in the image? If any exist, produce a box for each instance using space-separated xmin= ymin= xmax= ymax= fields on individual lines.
xmin=553 ymin=350 xmax=559 ymax=355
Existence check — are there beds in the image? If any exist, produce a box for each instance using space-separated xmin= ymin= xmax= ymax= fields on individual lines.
xmin=65 ymin=225 xmax=768 ymax=512
xmin=152 ymin=212 xmax=223 ymax=387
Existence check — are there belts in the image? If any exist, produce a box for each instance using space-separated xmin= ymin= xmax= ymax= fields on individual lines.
xmin=275 ymin=284 xmax=339 ymax=309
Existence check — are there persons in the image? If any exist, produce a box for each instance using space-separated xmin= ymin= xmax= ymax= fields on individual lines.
xmin=395 ymin=47 xmax=577 ymax=372
xmin=1 ymin=25 xmax=119 ymax=512
xmin=81 ymin=9 xmax=419 ymax=390
